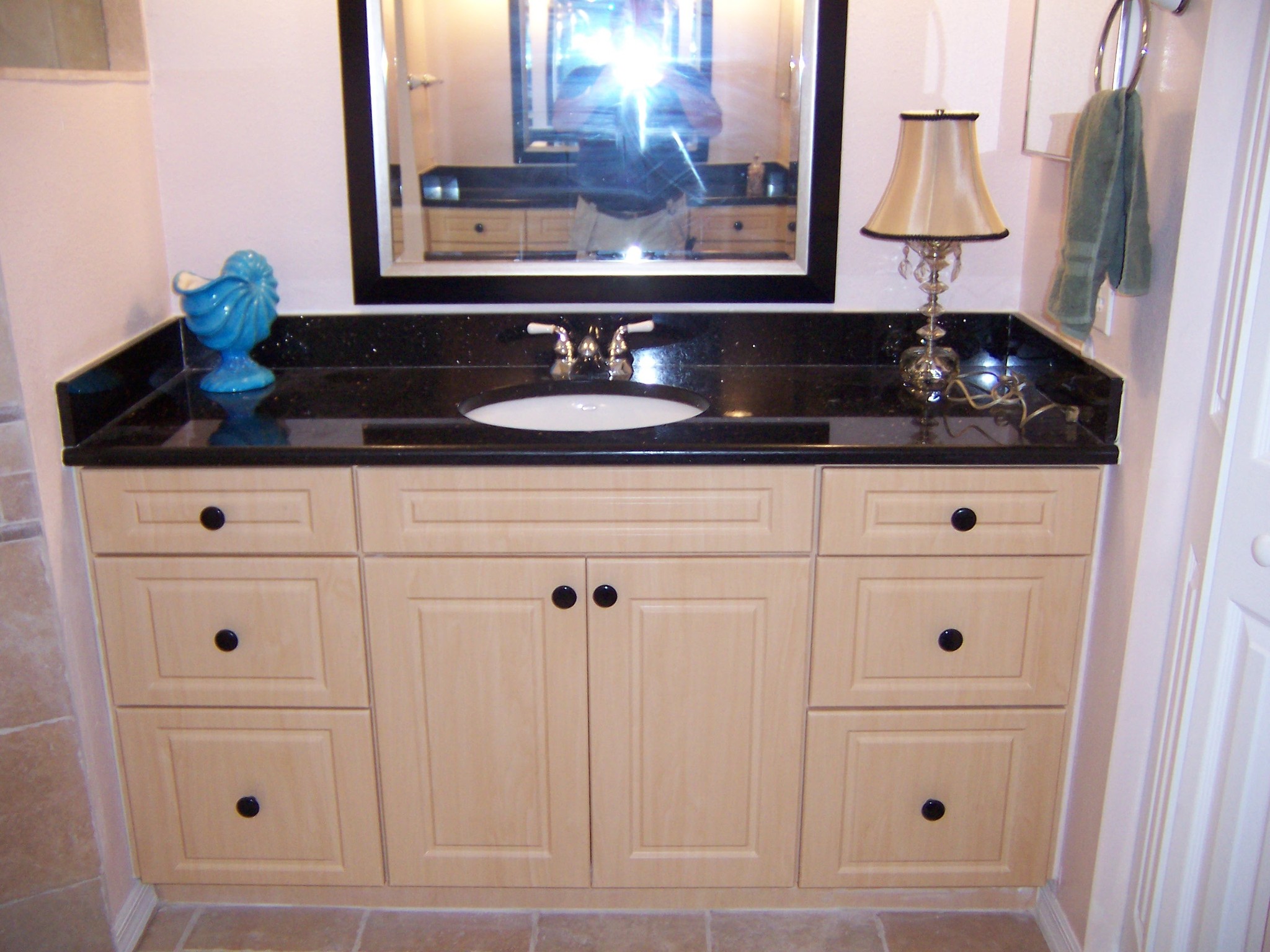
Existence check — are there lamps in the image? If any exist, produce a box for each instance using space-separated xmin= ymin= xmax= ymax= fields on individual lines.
xmin=859 ymin=110 xmax=1009 ymax=389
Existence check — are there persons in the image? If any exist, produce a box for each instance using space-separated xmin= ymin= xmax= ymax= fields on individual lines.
xmin=551 ymin=0 xmax=724 ymax=260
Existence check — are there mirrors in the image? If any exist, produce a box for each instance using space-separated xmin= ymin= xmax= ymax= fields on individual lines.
xmin=1020 ymin=0 xmax=1131 ymax=163
xmin=339 ymin=0 xmax=849 ymax=305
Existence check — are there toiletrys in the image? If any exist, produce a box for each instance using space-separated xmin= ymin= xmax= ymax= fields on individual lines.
xmin=745 ymin=153 xmax=766 ymax=195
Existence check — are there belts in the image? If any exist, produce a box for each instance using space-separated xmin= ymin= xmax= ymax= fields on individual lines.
xmin=579 ymin=190 xmax=686 ymax=220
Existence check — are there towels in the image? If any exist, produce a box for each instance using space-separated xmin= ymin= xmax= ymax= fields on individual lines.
xmin=1042 ymin=84 xmax=1152 ymax=342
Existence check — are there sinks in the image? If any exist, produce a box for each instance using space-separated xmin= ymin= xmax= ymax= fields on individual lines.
xmin=457 ymin=377 xmax=711 ymax=431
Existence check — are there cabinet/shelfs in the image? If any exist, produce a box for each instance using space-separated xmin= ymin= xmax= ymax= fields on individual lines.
xmin=796 ymin=461 xmax=1100 ymax=905
xmin=687 ymin=204 xmax=797 ymax=259
xmin=353 ymin=463 xmax=821 ymax=906
xmin=76 ymin=466 xmax=386 ymax=908
xmin=426 ymin=207 xmax=575 ymax=258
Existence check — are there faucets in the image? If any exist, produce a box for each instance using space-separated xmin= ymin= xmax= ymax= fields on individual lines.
xmin=575 ymin=335 xmax=606 ymax=381
